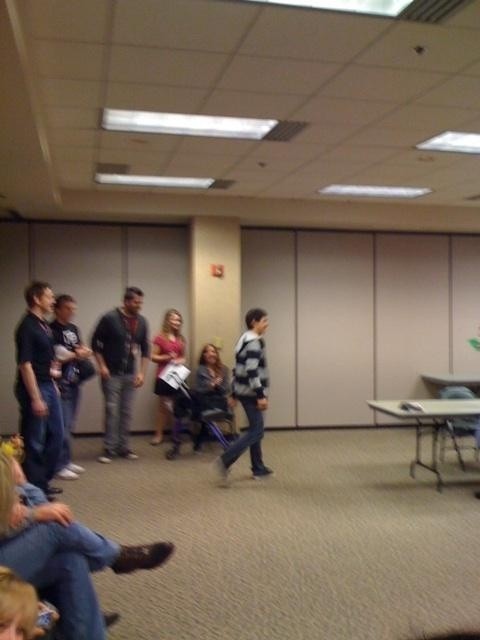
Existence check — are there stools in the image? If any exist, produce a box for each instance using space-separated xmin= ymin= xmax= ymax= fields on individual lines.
xmin=201 ymin=407 xmax=241 ymax=447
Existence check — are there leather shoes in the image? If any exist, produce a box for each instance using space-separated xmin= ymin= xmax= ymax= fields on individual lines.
xmin=48 ymin=486 xmax=64 ymax=495
xmin=110 ymin=539 xmax=175 ymax=577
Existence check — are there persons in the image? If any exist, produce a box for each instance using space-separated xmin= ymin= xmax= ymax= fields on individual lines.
xmin=0 ymin=432 xmax=175 ymax=639
xmin=149 ymin=307 xmax=188 ymax=445
xmin=14 ymin=279 xmax=65 ymax=505
xmin=205 ymin=308 xmax=276 ymax=488
xmin=48 ymin=294 xmax=93 ymax=479
xmin=87 ymin=285 xmax=151 ymax=464
xmin=189 ymin=342 xmax=235 ymax=453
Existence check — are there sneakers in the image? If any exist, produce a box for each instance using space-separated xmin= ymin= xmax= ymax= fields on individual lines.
xmin=148 ymin=435 xmax=165 ymax=448
xmin=96 ymin=452 xmax=113 ymax=464
xmin=122 ymin=450 xmax=140 ymax=460
xmin=253 ymin=467 xmax=276 ymax=480
xmin=210 ymin=457 xmax=232 ymax=489
xmin=65 ymin=461 xmax=86 ymax=474
xmin=57 ymin=466 xmax=80 ymax=480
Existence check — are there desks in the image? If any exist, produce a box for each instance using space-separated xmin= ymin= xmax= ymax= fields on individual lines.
xmin=365 ymin=396 xmax=480 ymax=495
xmin=421 ymin=372 xmax=480 ymax=388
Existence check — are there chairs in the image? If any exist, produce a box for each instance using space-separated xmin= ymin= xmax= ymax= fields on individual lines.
xmin=436 ymin=384 xmax=480 ymax=473
